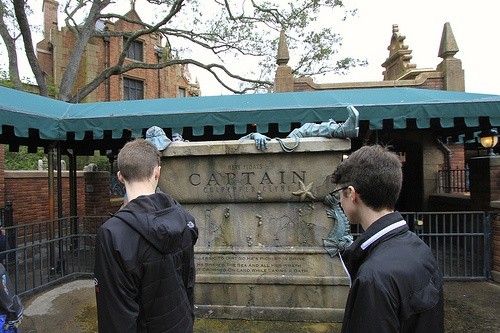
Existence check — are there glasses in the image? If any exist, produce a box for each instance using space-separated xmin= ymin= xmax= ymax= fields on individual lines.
xmin=327 ymin=185 xmax=357 ymax=201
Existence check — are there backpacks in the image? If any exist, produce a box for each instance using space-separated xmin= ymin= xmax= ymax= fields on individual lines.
xmin=0 ymin=263 xmax=23 ymax=332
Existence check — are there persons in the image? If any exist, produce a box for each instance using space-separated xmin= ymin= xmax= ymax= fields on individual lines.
xmin=238 ymin=104 xmax=360 ymax=150
xmin=0 ymin=261 xmax=25 ymax=333
xmin=94 ymin=140 xmax=199 ymax=333
xmin=329 ymin=144 xmax=445 ymax=333
